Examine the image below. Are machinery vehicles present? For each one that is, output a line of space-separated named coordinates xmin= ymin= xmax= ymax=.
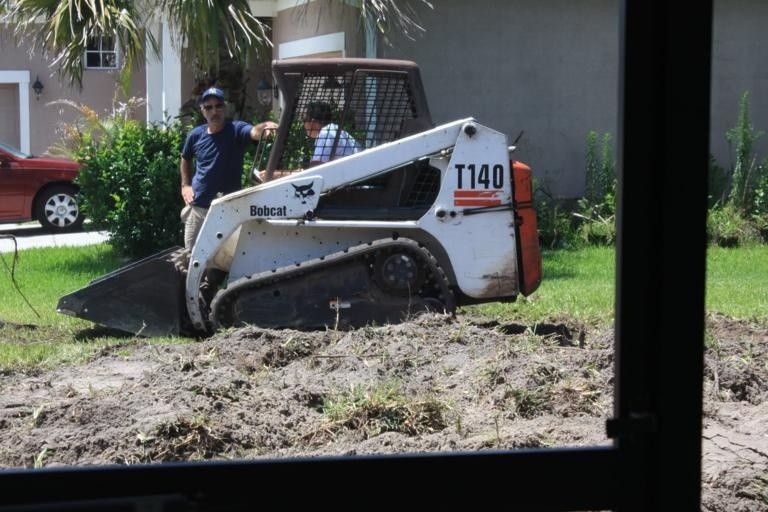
xmin=56 ymin=58 xmax=542 ymax=339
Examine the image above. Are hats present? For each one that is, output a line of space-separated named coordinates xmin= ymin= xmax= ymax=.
xmin=200 ymin=87 xmax=224 ymax=100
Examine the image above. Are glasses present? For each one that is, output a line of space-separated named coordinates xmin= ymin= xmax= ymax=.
xmin=202 ymin=103 xmax=225 ymax=111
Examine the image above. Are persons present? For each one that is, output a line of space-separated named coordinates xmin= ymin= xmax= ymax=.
xmin=259 ymin=102 xmax=364 ymax=179
xmin=180 ymin=87 xmax=279 ymax=251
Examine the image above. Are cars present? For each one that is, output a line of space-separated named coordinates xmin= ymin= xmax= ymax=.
xmin=0 ymin=143 xmax=90 ymax=233
